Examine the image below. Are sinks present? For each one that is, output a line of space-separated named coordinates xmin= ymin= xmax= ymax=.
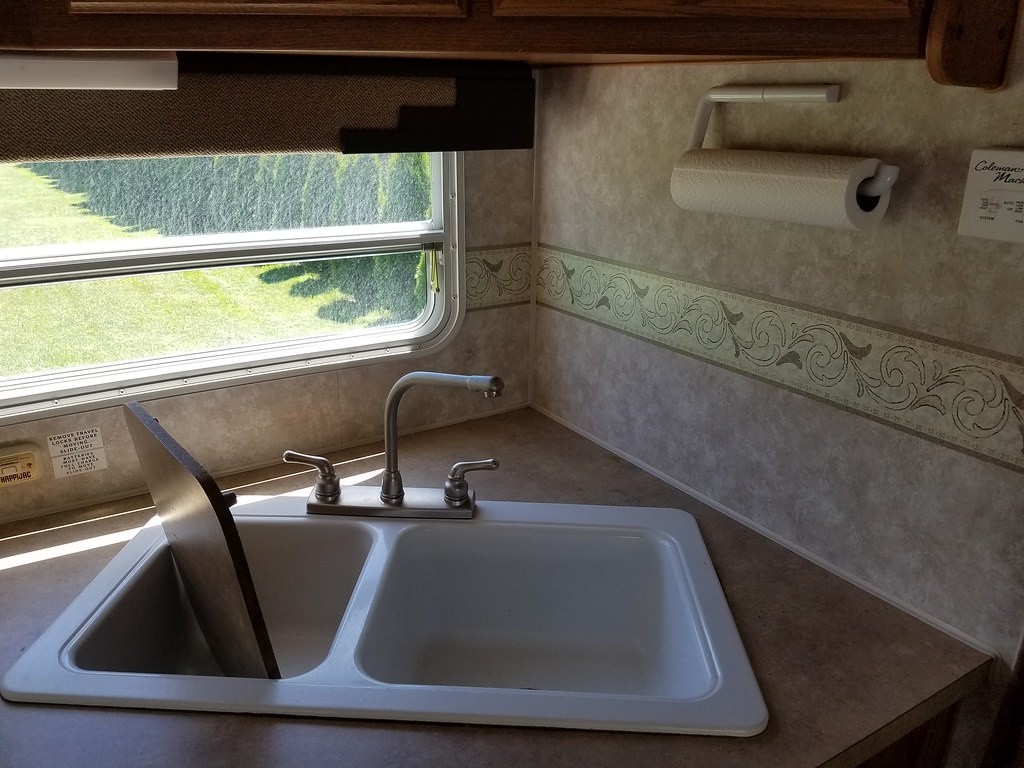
xmin=1 ymin=491 xmax=378 ymax=721
xmin=351 ymin=483 xmax=772 ymax=740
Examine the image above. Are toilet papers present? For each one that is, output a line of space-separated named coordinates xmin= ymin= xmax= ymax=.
xmin=668 ymin=147 xmax=895 ymax=234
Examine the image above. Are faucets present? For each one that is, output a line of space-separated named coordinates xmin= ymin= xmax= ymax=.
xmin=378 ymin=371 xmax=506 ymax=506
